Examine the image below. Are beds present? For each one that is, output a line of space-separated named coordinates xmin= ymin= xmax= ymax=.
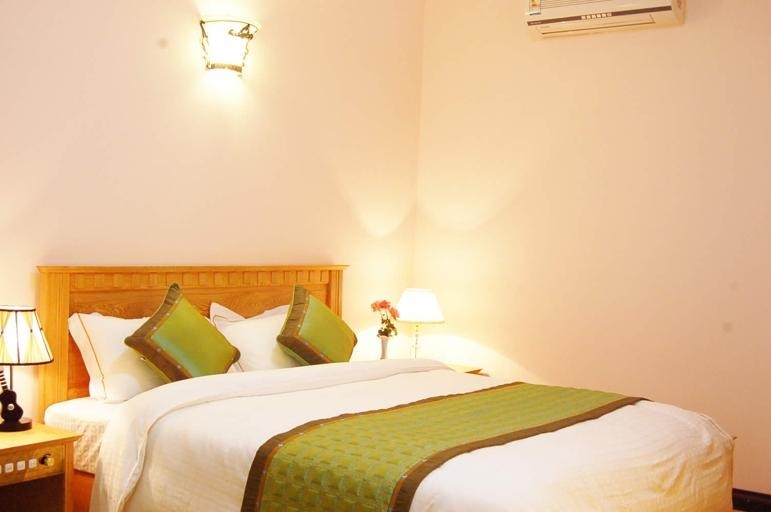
xmin=28 ymin=265 xmax=734 ymax=512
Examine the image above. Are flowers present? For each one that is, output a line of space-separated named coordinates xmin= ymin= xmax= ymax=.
xmin=371 ymin=300 xmax=400 ymax=338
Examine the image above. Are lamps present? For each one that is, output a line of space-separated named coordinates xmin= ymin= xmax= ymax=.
xmin=200 ymin=0 xmax=259 ymax=75
xmin=0 ymin=307 xmax=54 ymax=432
xmin=395 ymin=287 xmax=445 ymax=359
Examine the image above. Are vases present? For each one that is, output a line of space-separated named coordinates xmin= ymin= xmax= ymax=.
xmin=380 ymin=335 xmax=389 ymax=359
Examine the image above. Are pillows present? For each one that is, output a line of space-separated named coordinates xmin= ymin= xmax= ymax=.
xmin=277 ymin=285 xmax=358 ymax=366
xmin=68 ymin=312 xmax=240 ymax=402
xmin=123 ymin=281 xmax=240 ymax=385
xmin=208 ymin=301 xmax=293 ymax=373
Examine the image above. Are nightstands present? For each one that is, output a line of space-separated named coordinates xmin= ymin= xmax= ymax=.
xmin=0 ymin=421 xmax=82 ymax=512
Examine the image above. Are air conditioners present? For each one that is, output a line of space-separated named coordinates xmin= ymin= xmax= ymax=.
xmin=524 ymin=1 xmax=686 ymax=41
